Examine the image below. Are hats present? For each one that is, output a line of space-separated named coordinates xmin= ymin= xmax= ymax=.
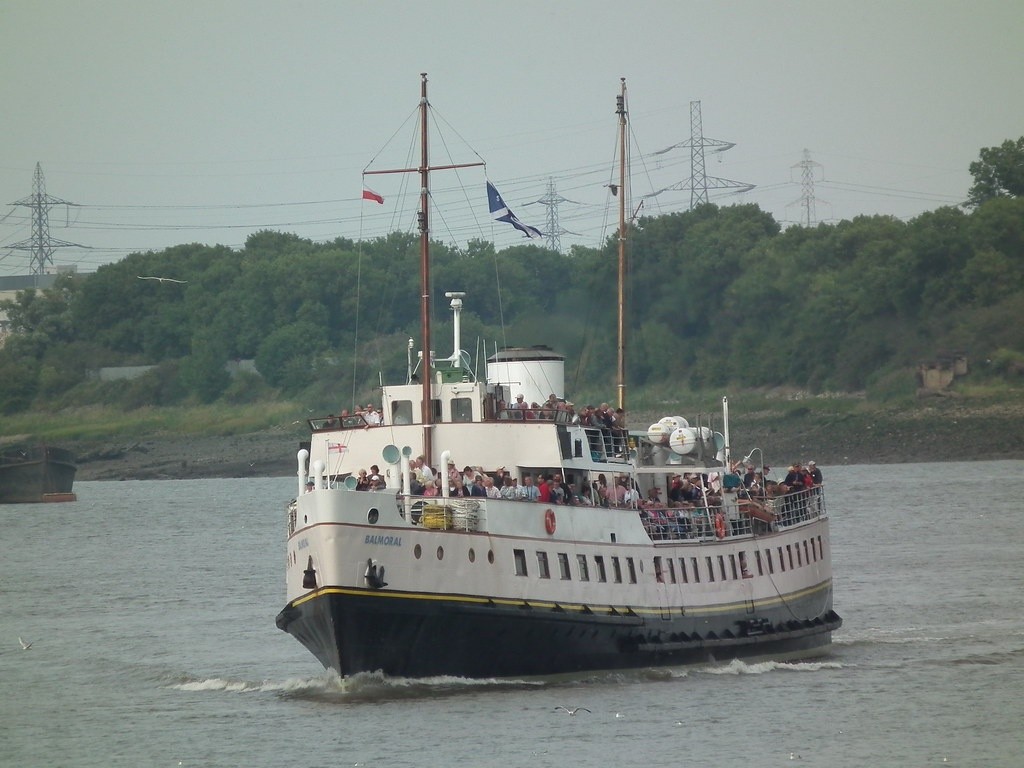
xmin=672 ymin=472 xmax=681 ymax=478
xmin=515 ymin=393 xmax=523 ymax=398
xmin=566 ymin=402 xmax=574 ymax=407
xmin=448 ymin=460 xmax=454 ymax=465
xmin=512 ymin=476 xmax=517 ymax=481
xmin=808 ymin=461 xmax=816 ymax=465
xmin=616 ymin=408 xmax=623 ymax=413
xmin=305 ymin=481 xmax=314 ymax=486
xmin=764 ymin=465 xmax=770 ymax=471
xmin=371 ymin=476 xmax=379 ymax=480
xmin=497 ymin=465 xmax=505 ymax=471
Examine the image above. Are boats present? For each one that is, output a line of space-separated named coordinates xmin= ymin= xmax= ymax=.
xmin=0 ymin=444 xmax=77 ymax=504
xmin=275 ymin=72 xmax=843 ymax=679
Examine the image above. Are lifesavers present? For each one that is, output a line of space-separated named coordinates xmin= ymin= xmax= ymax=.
xmin=546 ymin=509 xmax=556 ymax=534
xmin=715 ymin=513 xmax=725 ymax=539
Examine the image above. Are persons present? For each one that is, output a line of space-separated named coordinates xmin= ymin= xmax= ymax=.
xmin=638 ymin=461 xmax=823 ymax=540
xmin=356 ymin=465 xmax=386 ymax=492
xmin=409 ymin=456 xmax=442 ymax=496
xmin=323 ymin=404 xmax=383 ymax=430
xmin=498 ymin=393 xmax=625 ymax=431
xmin=448 ymin=460 xmax=639 ymax=509
xmin=305 ymin=482 xmax=314 ymax=492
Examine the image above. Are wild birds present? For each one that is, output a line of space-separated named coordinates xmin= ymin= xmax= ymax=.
xmin=18 ymin=637 xmax=33 ymax=651
xmin=603 ymin=183 xmax=622 ymax=196
xmin=675 ymin=721 xmax=685 ymax=725
xmin=554 ymin=704 xmax=592 ymax=716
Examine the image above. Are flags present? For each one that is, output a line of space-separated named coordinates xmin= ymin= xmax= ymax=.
xmin=363 ymin=183 xmax=384 ymax=204
xmin=487 ymin=178 xmax=542 ymax=238
